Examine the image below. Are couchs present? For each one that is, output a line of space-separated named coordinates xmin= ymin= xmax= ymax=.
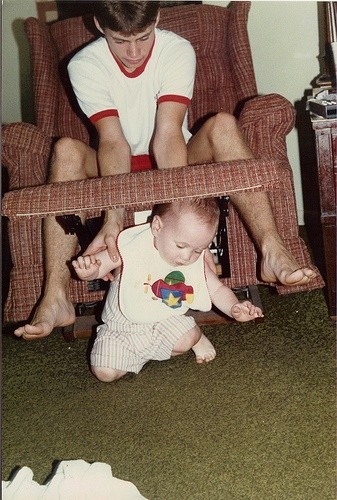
xmin=0 ymin=0 xmax=326 ymax=343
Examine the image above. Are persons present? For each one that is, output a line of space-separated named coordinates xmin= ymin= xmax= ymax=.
xmin=72 ymin=197 xmax=265 ymax=382
xmin=13 ymin=0 xmax=317 ymax=339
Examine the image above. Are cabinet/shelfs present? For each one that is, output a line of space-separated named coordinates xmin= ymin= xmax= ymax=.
xmin=296 ymin=112 xmax=337 ymax=316
